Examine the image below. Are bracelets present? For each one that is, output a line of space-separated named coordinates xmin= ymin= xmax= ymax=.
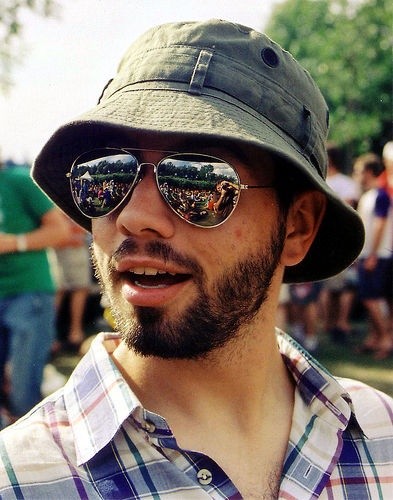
xmin=16 ymin=233 xmax=28 ymax=253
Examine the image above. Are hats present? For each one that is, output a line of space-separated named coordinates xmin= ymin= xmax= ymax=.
xmin=30 ymin=16 xmax=368 ymax=281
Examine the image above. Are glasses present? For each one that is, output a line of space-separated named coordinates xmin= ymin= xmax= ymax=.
xmin=66 ymin=146 xmax=301 ymax=228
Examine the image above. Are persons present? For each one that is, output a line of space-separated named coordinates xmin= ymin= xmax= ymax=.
xmin=277 ymin=139 xmax=393 ymax=359
xmin=44 ymin=213 xmax=94 ymax=357
xmin=0 ymin=18 xmax=393 ymax=500
xmin=71 ymin=172 xmax=239 ymax=226
xmin=0 ymin=158 xmax=71 ymax=422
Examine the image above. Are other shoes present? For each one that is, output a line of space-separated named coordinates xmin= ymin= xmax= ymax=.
xmin=301 ymin=327 xmax=392 ymax=360
